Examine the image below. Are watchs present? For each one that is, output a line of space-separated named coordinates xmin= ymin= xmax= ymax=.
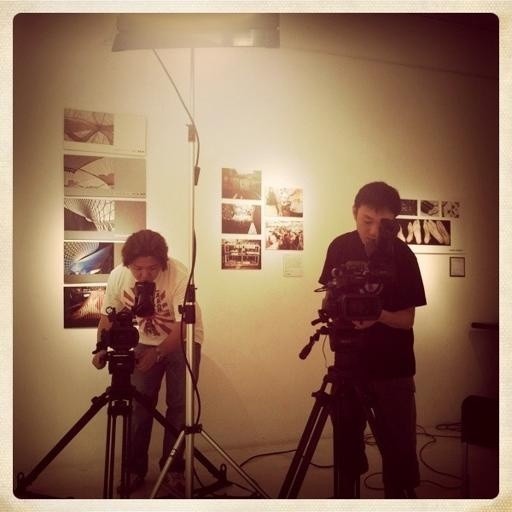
xmin=155 ymin=347 xmax=163 ymax=363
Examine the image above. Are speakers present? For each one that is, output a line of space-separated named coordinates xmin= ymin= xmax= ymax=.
xmin=461 ymin=395 xmax=499 ymax=444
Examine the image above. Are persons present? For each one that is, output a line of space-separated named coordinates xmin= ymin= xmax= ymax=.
xmin=315 ymin=175 xmax=428 ymax=499
xmin=89 ymin=228 xmax=203 ymax=495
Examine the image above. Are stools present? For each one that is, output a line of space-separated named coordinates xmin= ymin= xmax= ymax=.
xmin=461 ymin=396 xmax=499 ymax=498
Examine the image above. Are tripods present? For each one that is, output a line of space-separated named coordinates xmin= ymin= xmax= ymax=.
xmin=278 ymin=323 xmax=418 ymax=499
xmin=14 ymin=350 xmax=231 ymax=497
xmin=150 ymin=46 xmax=269 ymax=499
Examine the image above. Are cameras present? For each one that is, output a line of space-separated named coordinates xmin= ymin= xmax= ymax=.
xmin=89 ymin=280 xmax=157 ymax=353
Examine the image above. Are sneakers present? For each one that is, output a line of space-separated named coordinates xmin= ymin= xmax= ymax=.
xmin=116 ymin=472 xmax=144 ymax=493
xmin=164 ymin=473 xmax=185 ymax=495
